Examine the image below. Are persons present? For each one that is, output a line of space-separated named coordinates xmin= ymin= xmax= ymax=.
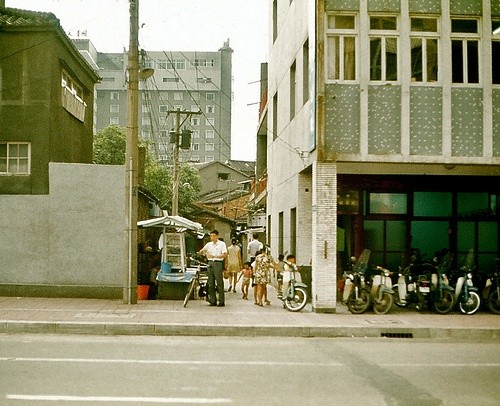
xmin=284 ymin=255 xmax=298 ymax=271
xmin=247 ymin=232 xmax=263 ymax=263
xmin=225 ymin=237 xmax=242 ymax=293
xmin=273 ymin=254 xmax=286 ymax=292
xmin=433 ymin=248 xmax=449 ymax=266
xmin=235 ymin=261 xmax=253 ymax=300
xmin=411 ymin=248 xmax=421 ymax=274
xmin=251 ymin=245 xmax=283 ymax=305
xmin=200 ymin=230 xmax=228 ymax=307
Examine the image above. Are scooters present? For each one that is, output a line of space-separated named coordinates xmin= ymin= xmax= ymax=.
xmin=278 ymin=260 xmax=308 ymax=312
xmin=342 ymin=256 xmax=500 ymax=316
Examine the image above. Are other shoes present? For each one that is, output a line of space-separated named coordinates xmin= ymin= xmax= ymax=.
xmin=218 ymin=303 xmax=225 ymax=307
xmin=209 ymin=303 xmax=217 ymax=306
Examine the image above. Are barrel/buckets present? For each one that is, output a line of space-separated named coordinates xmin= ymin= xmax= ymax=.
xmin=161 ymin=261 xmax=172 ymax=274
xmin=137 ymin=285 xmax=150 ymax=300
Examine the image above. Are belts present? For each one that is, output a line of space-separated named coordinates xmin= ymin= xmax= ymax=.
xmin=208 ymin=260 xmax=222 ymax=263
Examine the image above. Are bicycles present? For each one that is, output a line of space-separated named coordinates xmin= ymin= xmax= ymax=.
xmin=183 ymin=257 xmax=221 ymax=308
